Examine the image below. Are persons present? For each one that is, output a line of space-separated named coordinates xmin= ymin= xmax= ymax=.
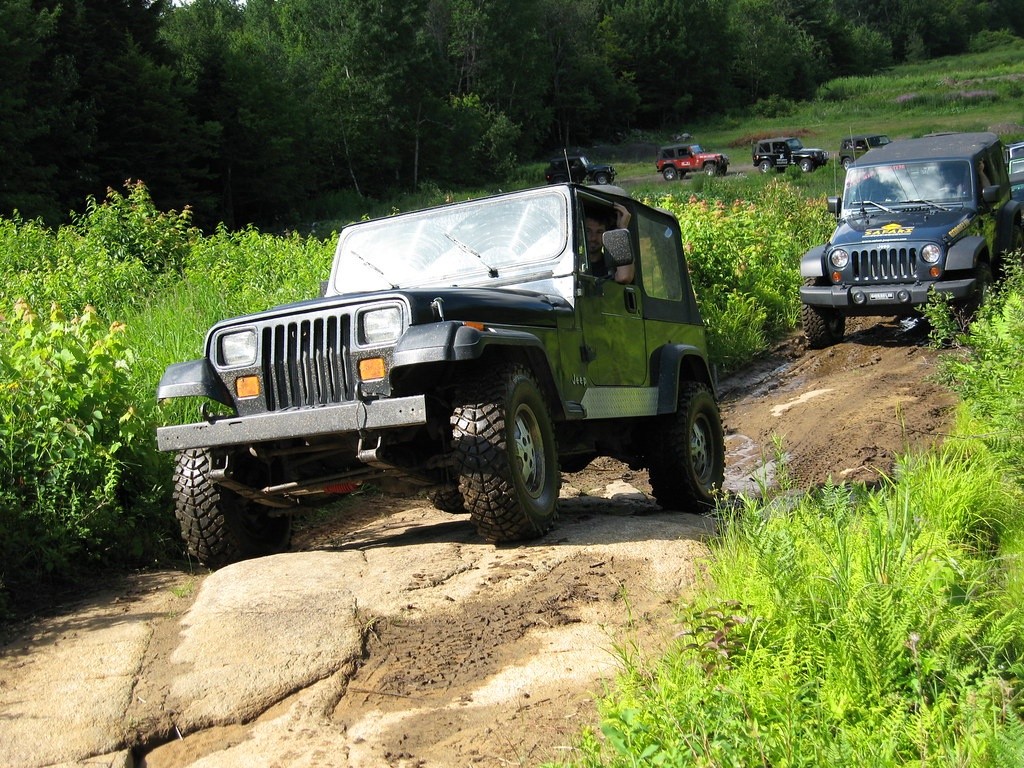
xmin=583 ymin=201 xmax=637 ymax=285
xmin=976 ymin=159 xmax=991 ymax=187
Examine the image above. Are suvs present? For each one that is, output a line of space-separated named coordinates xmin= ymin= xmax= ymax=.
xmin=751 ymin=137 xmax=830 ymax=175
xmin=655 ymin=144 xmax=731 ymax=181
xmin=544 ymin=155 xmax=618 ymax=185
xmin=153 ymin=179 xmax=730 ymax=571
xmin=838 ymin=134 xmax=894 ymax=174
xmin=920 ymin=131 xmax=1024 ymax=225
xmin=798 ymin=132 xmax=1024 ymax=348
